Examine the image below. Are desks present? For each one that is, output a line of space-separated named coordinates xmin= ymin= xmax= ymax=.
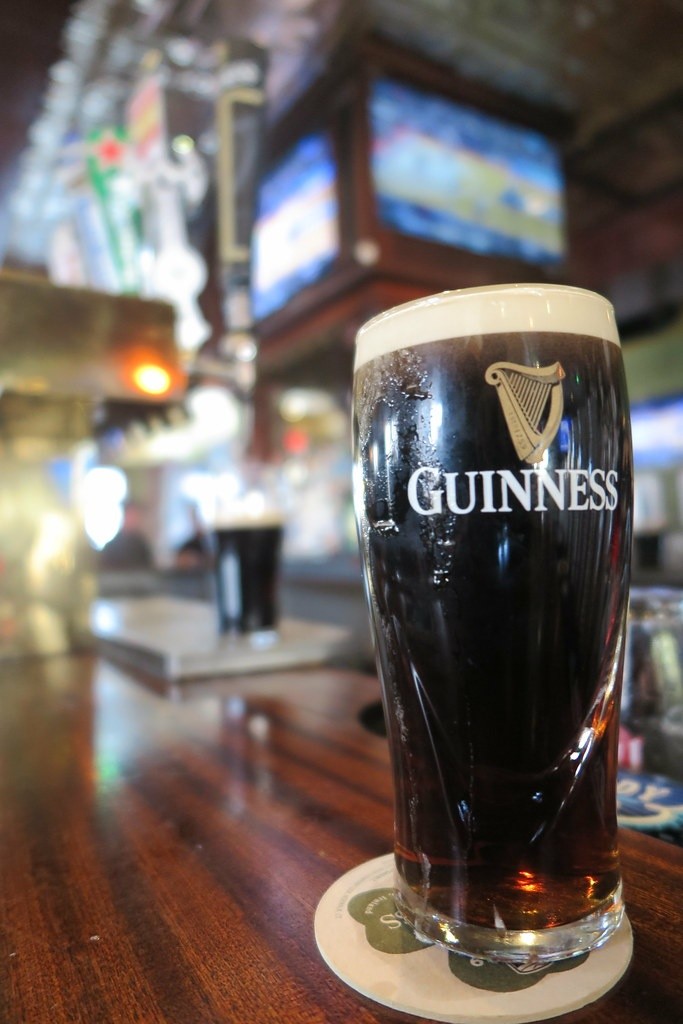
xmin=1 ymin=655 xmax=683 ymax=1024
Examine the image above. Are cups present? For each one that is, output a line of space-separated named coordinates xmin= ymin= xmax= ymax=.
xmin=352 ymin=280 xmax=636 ymax=970
xmin=200 ymin=465 xmax=287 ymax=647
